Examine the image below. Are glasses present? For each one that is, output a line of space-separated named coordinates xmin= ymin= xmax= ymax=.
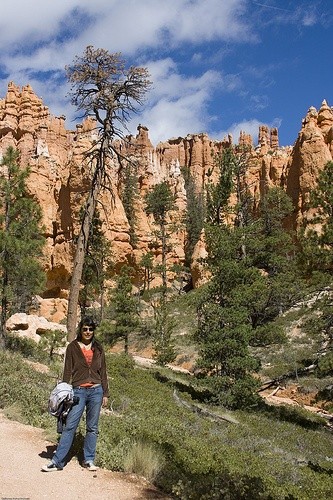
xmin=81 ymin=327 xmax=94 ymax=333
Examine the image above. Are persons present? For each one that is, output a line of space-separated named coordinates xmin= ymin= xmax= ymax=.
xmin=40 ymin=317 xmax=110 ymax=472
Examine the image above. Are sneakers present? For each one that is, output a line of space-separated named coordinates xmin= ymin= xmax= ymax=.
xmin=42 ymin=461 xmax=64 ymax=472
xmin=84 ymin=461 xmax=98 ymax=471
